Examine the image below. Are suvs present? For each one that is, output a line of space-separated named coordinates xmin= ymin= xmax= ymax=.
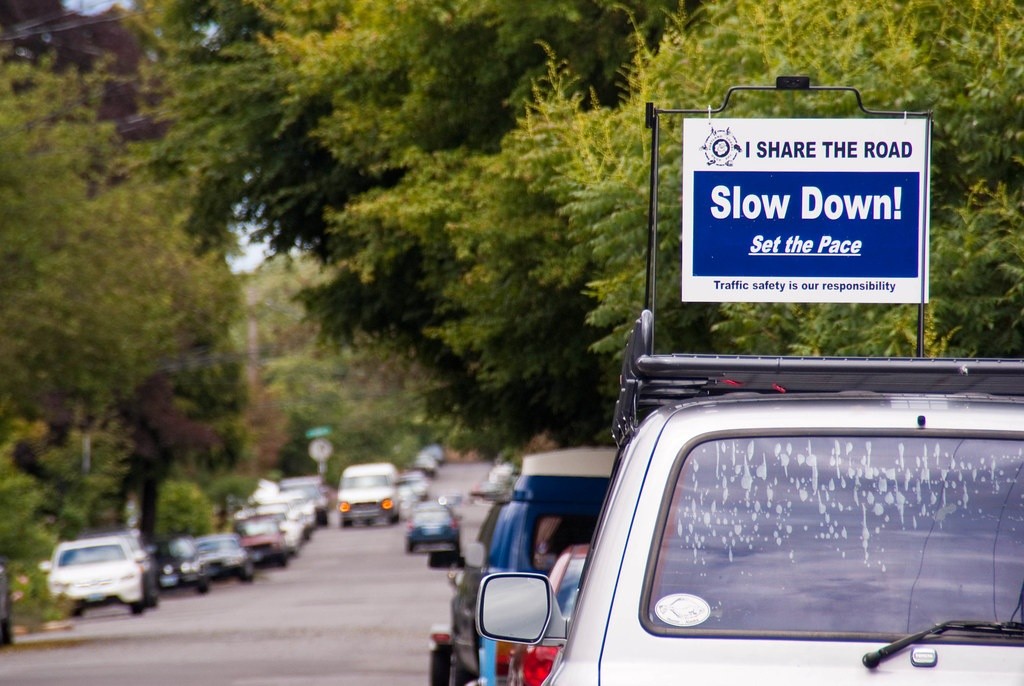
xmin=253 ymin=478 xmax=327 ymax=557
xmin=39 ymin=527 xmax=157 ymax=617
xmin=235 ymin=518 xmax=288 ymax=568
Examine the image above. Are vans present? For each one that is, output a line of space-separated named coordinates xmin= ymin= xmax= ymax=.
xmin=449 ymin=446 xmax=619 ymax=686
xmin=336 ymin=463 xmax=399 ymax=527
xmin=478 ymin=307 xmax=1024 ymax=686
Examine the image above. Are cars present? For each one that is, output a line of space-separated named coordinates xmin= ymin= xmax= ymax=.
xmin=405 ymin=502 xmax=458 ymax=552
xmin=146 ymin=533 xmax=208 ymax=593
xmin=193 ymin=535 xmax=253 ymax=582
xmin=398 ymin=442 xmax=443 ymax=518
xmin=507 ymin=544 xmax=589 ymax=686
